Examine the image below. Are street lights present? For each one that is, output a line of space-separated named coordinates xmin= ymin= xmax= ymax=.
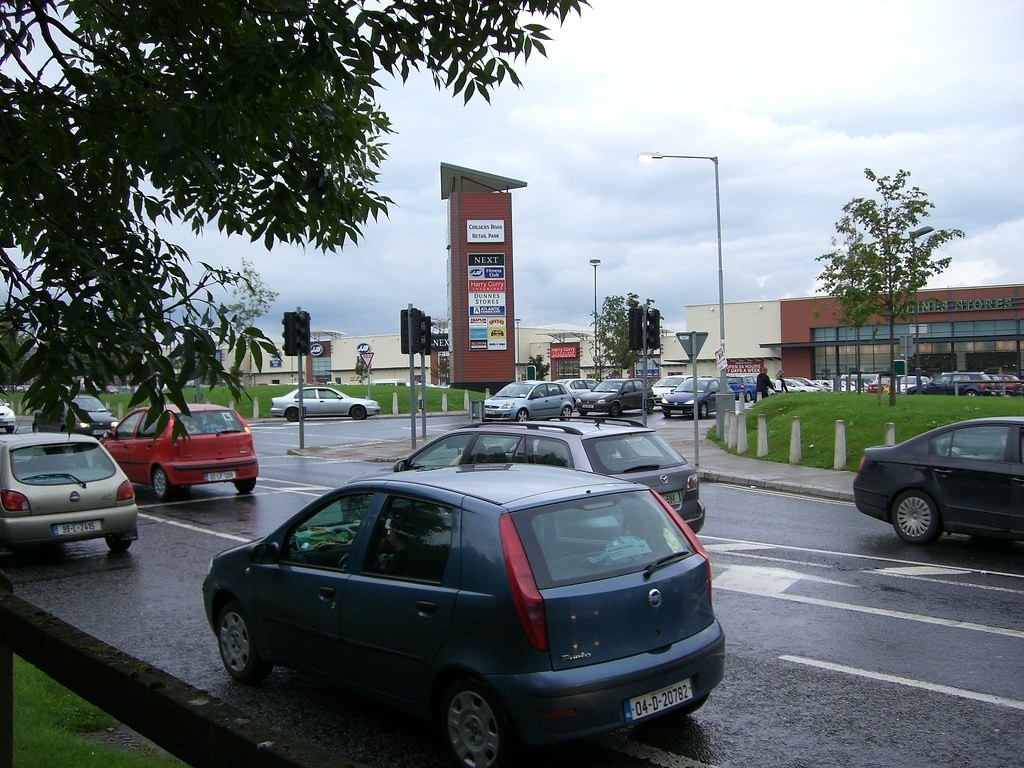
xmin=909 ymin=227 xmax=934 ymax=391
xmin=589 ymin=259 xmax=601 ymax=381
xmin=515 ymin=318 xmax=521 ymax=381
xmin=637 ymin=152 xmax=726 ymax=393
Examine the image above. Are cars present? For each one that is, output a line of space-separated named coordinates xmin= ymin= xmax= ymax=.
xmin=483 ymin=379 xmax=574 ymax=423
xmin=99 ymin=404 xmax=258 ymax=501
xmin=0 ymin=432 xmax=138 ymax=550
xmin=907 ymin=371 xmax=996 ymax=396
xmin=270 ymin=387 xmax=381 ymax=420
xmin=204 ymin=464 xmax=724 ymax=768
xmin=988 ymin=375 xmax=1024 ymax=396
xmin=0 ymin=398 xmax=16 ymax=432
xmin=771 ymin=375 xmax=930 ymax=396
xmin=3 ymin=380 xmax=140 ymax=393
xmin=853 ymin=416 xmax=1024 ymax=545
xmin=32 ymin=394 xmax=119 ymax=439
xmin=552 ymin=376 xmax=756 ymax=422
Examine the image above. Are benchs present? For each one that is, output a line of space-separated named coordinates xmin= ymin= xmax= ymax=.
xmin=940 ymin=438 xmax=961 ymax=456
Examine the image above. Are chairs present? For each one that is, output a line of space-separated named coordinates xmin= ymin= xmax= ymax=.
xmin=484 ymin=446 xmax=507 ymax=463
xmin=537 ymin=440 xmax=557 ymax=456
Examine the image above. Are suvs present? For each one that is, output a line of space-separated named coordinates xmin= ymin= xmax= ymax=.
xmin=341 ymin=415 xmax=705 ymax=543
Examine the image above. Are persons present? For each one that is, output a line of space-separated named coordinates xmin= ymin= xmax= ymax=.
xmin=377 ymin=516 xmax=415 ymax=576
xmin=773 ymin=370 xmax=789 ymax=394
xmin=754 ymin=367 xmax=774 ymax=403
xmin=448 ymin=442 xmax=486 ymax=465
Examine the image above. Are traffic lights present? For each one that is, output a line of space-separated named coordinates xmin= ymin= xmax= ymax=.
xmin=646 ymin=308 xmax=660 ymax=350
xmin=296 ymin=311 xmax=310 ymax=354
